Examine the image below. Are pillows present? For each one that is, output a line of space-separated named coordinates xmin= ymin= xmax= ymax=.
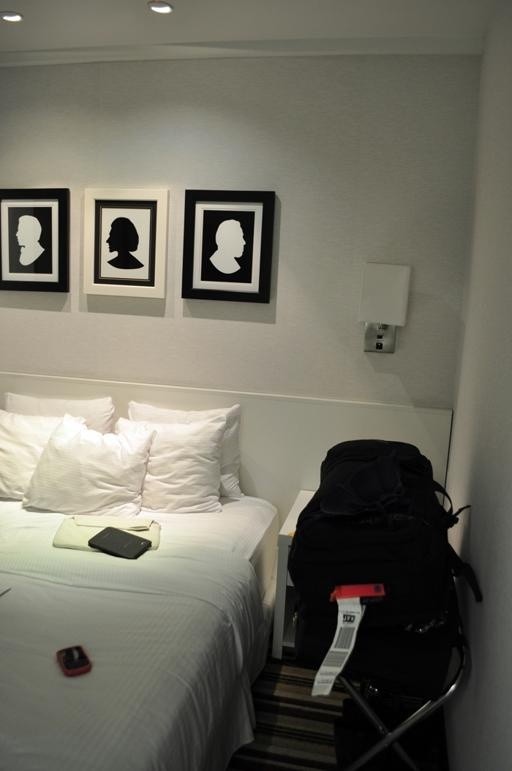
xmin=0 ymin=398 xmax=247 ymax=519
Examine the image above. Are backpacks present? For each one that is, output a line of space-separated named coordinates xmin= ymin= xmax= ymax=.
xmin=288 ymin=440 xmax=462 ymax=698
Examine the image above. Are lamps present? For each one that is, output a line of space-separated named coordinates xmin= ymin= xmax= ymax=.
xmin=357 ymin=262 xmax=413 ymax=354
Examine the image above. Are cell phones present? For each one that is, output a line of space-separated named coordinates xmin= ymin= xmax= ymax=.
xmin=57 ymin=646 xmax=92 ymax=676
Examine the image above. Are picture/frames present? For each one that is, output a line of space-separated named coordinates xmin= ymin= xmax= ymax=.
xmin=181 ymin=189 xmax=275 ymax=304
xmin=82 ymin=187 xmax=170 ymax=300
xmin=0 ymin=187 xmax=71 ymax=294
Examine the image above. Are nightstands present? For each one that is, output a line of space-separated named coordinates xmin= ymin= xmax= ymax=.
xmin=273 ymin=488 xmax=318 ymax=660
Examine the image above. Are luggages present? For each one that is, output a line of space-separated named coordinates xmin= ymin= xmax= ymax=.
xmin=333 ymin=682 xmax=448 ymax=771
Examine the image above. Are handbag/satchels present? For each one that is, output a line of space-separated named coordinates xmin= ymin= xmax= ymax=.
xmin=53 ymin=516 xmax=160 ymax=552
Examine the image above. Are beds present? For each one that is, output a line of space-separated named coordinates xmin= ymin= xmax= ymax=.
xmin=0 ymin=495 xmax=281 ymax=771
